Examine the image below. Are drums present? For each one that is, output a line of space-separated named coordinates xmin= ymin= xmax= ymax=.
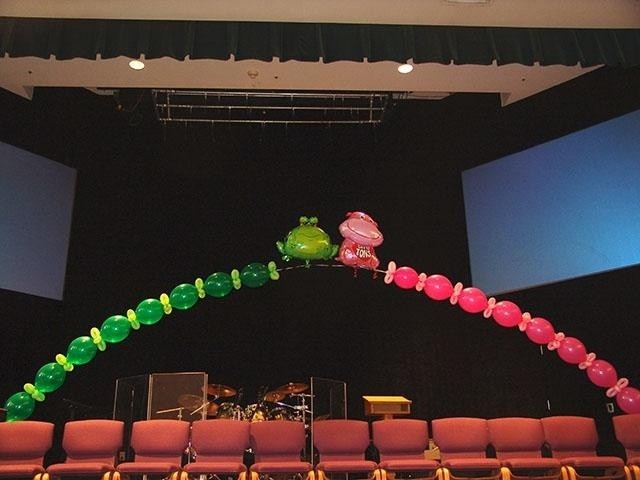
xmin=246 ymin=404 xmax=268 ymax=421
xmin=217 ymin=403 xmax=245 ymax=419
xmin=270 ymin=408 xmax=291 ymax=420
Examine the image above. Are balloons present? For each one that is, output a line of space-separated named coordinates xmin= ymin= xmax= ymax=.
xmin=275 ymin=216 xmax=339 ymax=268
xmin=2 ymin=262 xmax=279 ymax=424
xmin=384 ymin=261 xmax=640 ymax=413
xmin=334 ymin=211 xmax=384 ymax=280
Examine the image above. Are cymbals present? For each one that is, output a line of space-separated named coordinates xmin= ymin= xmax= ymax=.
xmin=201 ymin=384 xmax=236 ymax=397
xmin=279 ymin=384 xmax=308 ymax=392
xmin=179 ymin=395 xmax=218 ymax=416
xmin=263 ymin=392 xmax=285 ymax=401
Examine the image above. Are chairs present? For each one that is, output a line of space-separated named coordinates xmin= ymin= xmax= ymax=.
xmin=371 ymin=418 xmax=444 ymax=479
xmin=541 ymin=416 xmax=632 ymax=478
xmin=432 ymin=417 xmax=506 ymax=479
xmin=610 ymin=413 xmax=638 ymax=479
xmin=1 ymin=420 xmax=56 ymax=479
xmin=180 ymin=419 xmax=250 ymax=478
xmin=42 ymin=420 xmax=124 ymax=479
xmin=249 ymin=421 xmax=316 ymax=480
xmin=112 ymin=420 xmax=190 ymax=479
xmin=489 ymin=416 xmax=569 ymax=479
xmin=310 ymin=420 xmax=382 ymax=478
